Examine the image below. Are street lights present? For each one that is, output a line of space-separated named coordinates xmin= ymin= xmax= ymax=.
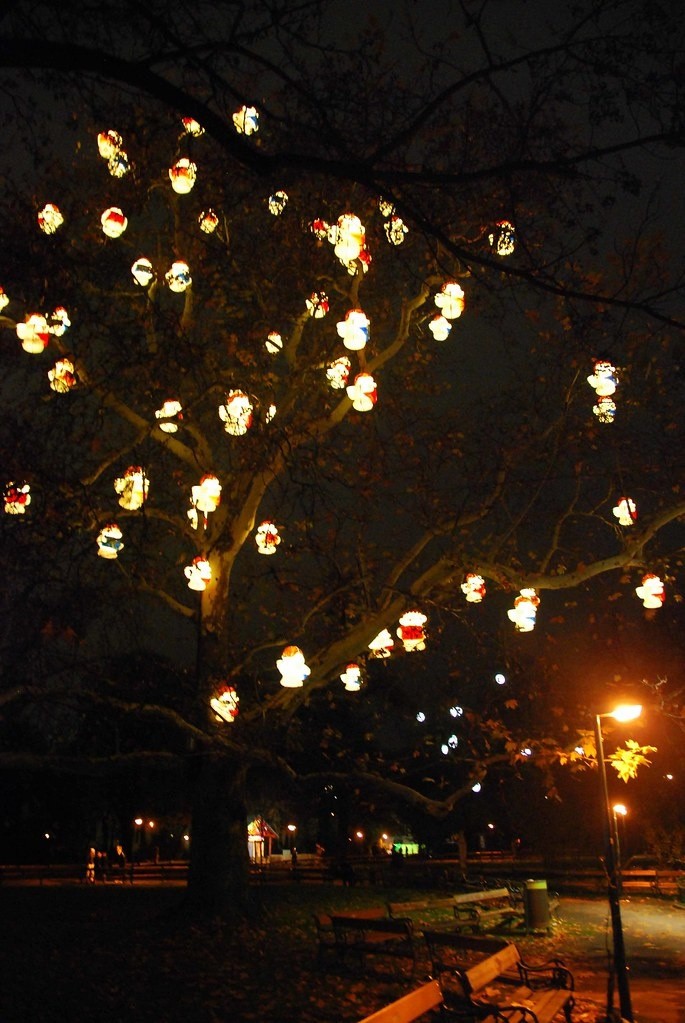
xmin=129 ymin=817 xmax=143 ymax=884
xmin=287 ymin=824 xmax=298 ymax=869
xmin=597 ymin=702 xmax=648 ymax=1023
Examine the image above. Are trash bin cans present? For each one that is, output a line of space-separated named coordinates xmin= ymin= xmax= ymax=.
xmin=522 ymin=879 xmax=553 ymax=928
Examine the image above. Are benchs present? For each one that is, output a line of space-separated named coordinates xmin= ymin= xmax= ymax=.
xmin=325 ymin=912 xmax=441 ymax=986
xmin=449 ymin=886 xmax=529 ymax=940
xmin=384 ymin=897 xmax=479 ymax=958
xmin=424 ymin=931 xmax=576 ymax=1023
